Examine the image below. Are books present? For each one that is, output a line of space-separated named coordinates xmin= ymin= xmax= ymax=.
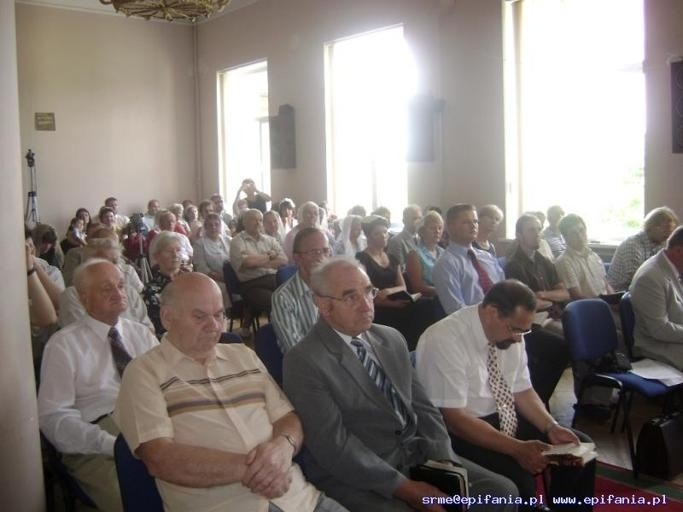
xmin=411 ymin=458 xmax=470 ymax=511
xmin=542 ymin=440 xmax=596 ymax=477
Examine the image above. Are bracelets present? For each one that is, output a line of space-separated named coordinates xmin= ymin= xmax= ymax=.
xmin=279 ymin=431 xmax=297 ymax=452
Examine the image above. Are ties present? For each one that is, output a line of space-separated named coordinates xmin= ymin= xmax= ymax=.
xmin=467 ymin=249 xmax=493 ymax=296
xmin=487 ymin=342 xmax=519 ymax=439
xmin=108 ymin=327 xmax=132 ymax=380
xmin=350 ymin=336 xmax=409 ymax=431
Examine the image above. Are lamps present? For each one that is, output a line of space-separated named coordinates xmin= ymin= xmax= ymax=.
xmin=99 ymin=1 xmax=231 ymax=25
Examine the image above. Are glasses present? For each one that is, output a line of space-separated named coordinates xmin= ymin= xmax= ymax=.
xmin=318 ymin=287 xmax=378 ymax=306
xmin=504 ymin=321 xmax=531 ymax=338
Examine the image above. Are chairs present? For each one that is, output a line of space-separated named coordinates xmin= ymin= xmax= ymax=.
xmin=112 ymin=260 xmax=682 ymax=511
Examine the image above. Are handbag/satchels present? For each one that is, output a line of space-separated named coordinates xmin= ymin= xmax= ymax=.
xmin=599 ymin=353 xmax=631 ymax=374
xmin=636 ymin=411 xmax=682 ymax=480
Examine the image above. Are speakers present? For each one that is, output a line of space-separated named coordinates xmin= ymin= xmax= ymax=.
xmin=670 ymin=60 xmax=683 ymax=153
xmin=270 ymin=104 xmax=296 ymax=169
xmin=407 ymin=89 xmax=435 ymax=161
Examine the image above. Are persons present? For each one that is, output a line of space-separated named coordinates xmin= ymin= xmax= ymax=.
xmin=113 ymin=271 xmax=350 ymax=512
xmin=36 ymin=261 xmax=161 ymax=511
xmin=414 ymin=280 xmax=595 ymax=511
xmin=21 ymin=176 xmax=682 ymax=379
xmin=282 ymin=258 xmax=519 ymax=511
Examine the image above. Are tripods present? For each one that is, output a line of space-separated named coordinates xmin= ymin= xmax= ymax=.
xmin=24 ymin=149 xmax=38 ymax=226
xmin=133 ymin=233 xmax=154 ymax=284
